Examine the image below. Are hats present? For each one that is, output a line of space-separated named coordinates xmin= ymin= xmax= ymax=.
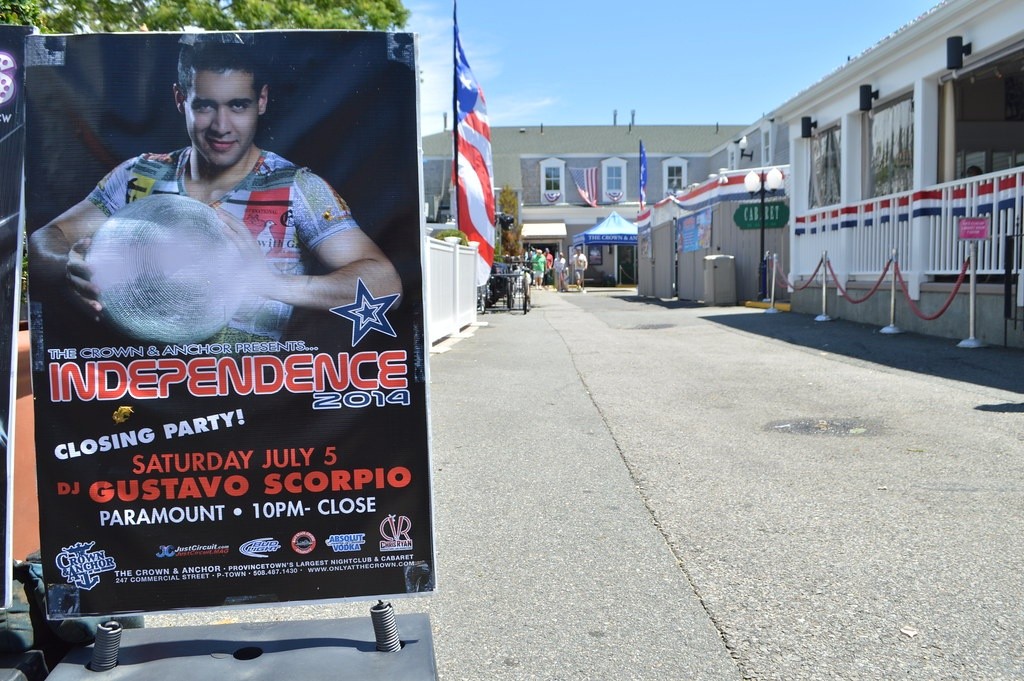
xmin=576 ymin=246 xmax=581 ymax=250
xmin=545 ymin=248 xmax=549 ymax=252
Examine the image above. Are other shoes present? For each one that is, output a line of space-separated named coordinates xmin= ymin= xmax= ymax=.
xmin=535 ymin=286 xmax=542 ymax=290
xmin=577 ymin=286 xmax=583 ymax=292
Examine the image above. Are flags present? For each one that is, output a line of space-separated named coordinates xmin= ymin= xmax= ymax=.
xmin=446 ymin=1 xmax=499 ymax=269
xmin=567 ymin=166 xmax=600 ymax=209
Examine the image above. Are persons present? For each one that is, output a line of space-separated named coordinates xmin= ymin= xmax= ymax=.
xmin=571 ymin=247 xmax=588 ymax=292
xmin=529 ymin=246 xmax=553 ymax=290
xmin=554 ymin=252 xmax=566 ymax=292
xmin=27 ymin=35 xmax=406 ymax=316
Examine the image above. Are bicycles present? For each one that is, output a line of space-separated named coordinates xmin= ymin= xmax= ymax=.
xmin=478 ymin=286 xmax=487 ymax=314
xmin=522 ymin=260 xmax=538 ymax=314
xmin=501 ymin=261 xmax=520 ymax=308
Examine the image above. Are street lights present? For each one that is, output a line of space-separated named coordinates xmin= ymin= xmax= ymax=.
xmin=744 ymin=169 xmax=783 ymax=302
